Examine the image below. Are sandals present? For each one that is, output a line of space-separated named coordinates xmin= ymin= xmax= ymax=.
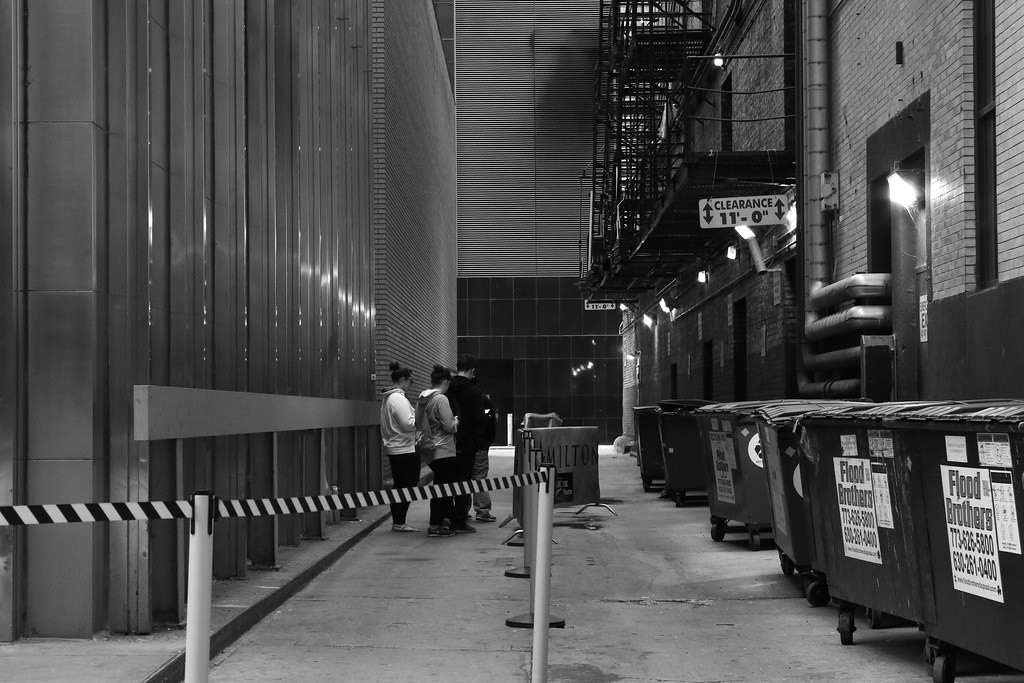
xmin=392 ymin=522 xmax=421 ymax=532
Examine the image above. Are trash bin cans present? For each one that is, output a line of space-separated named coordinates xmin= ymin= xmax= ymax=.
xmin=690 ymin=396 xmax=861 ymax=530
xmin=656 ymin=399 xmax=721 ymax=504
xmin=798 ymin=398 xmax=1024 ymax=673
xmin=750 ymin=401 xmax=912 ymax=572
xmin=631 ymin=405 xmax=664 ymax=493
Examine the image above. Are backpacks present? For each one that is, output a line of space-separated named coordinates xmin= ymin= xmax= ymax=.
xmin=414 ymin=393 xmax=454 ymax=453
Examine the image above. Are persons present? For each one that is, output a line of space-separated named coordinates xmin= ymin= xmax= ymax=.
xmin=379 ymin=361 xmax=422 ymax=531
xmin=414 ymin=363 xmax=497 ymax=537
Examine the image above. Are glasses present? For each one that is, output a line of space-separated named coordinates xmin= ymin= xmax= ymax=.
xmin=403 ymin=376 xmax=413 ymax=385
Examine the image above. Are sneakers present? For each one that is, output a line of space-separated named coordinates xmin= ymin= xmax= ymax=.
xmin=451 ymin=522 xmax=476 ymax=533
xmin=466 ymin=515 xmax=472 ymax=520
xmin=427 ymin=527 xmax=456 ymax=537
xmin=476 ymin=512 xmax=497 ymax=522
